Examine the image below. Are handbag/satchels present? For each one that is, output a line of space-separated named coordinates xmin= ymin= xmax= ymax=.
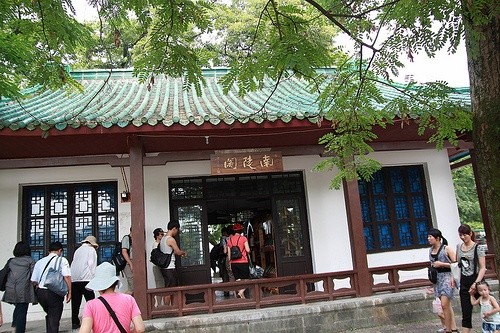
xmin=44 ymin=257 xmax=68 ymax=296
xmin=428 ymin=267 xmax=437 ymax=283
xmin=0 ymin=258 xmax=13 ymax=291
xmin=112 ymin=235 xmax=132 ymax=270
xmin=150 ymin=248 xmax=171 ymax=268
xmin=432 ymin=297 xmax=444 ymax=318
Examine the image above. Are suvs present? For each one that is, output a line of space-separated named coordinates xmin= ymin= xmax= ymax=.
xmin=474 ymin=239 xmax=488 ymax=251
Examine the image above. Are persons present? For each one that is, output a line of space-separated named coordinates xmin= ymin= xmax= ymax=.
xmin=456 ymin=224 xmax=486 ymax=333
xmin=1 ymin=241 xmax=38 ymax=333
xmin=78 ymin=262 xmax=145 ymax=333
xmin=70 ymin=236 xmax=99 ymax=329
xmin=123 ymin=227 xmax=134 ymax=295
xmin=469 ymin=281 xmax=500 ymax=333
xmin=152 ymin=228 xmax=168 ymax=306
xmin=218 ymin=223 xmax=252 ymax=299
xmin=427 ymin=228 xmax=460 ymax=333
xmin=159 ymin=219 xmax=187 ymax=306
xmin=30 ymin=241 xmax=72 ymax=333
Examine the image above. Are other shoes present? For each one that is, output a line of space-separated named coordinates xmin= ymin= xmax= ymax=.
xmin=237 ymin=292 xmax=245 ymax=299
xmin=437 ymin=327 xmax=446 ymax=333
xmin=72 ymin=324 xmax=81 ymax=329
xmin=447 ymin=330 xmax=460 ymax=333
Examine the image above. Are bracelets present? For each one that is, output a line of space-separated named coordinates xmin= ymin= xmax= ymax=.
xmin=474 ymin=282 xmax=476 ymax=285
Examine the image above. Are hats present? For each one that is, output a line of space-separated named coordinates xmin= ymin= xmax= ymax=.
xmin=81 ymin=236 xmax=99 ymax=250
xmin=84 ymin=262 xmax=122 ymax=291
xmin=233 ymin=223 xmax=243 ymax=230
xmin=153 ymin=228 xmax=168 ymax=233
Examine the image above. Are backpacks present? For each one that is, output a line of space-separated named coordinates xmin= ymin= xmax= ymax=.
xmin=231 ymin=236 xmax=245 ymax=260
